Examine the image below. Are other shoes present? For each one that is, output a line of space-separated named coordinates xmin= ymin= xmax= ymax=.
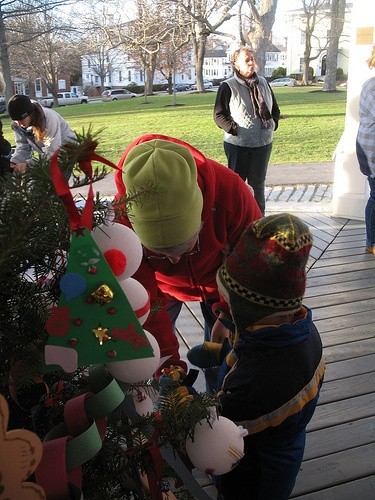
xmin=366 ymin=245 xmax=375 ymax=254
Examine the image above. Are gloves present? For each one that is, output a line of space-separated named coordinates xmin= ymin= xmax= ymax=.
xmin=187 ymin=342 xmax=224 ymax=368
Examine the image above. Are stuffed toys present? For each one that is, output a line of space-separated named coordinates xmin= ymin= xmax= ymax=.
xmin=91 ymin=221 xmax=160 ymax=385
xmin=185 ymin=417 xmax=248 ymax=476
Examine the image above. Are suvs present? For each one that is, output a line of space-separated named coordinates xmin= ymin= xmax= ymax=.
xmin=101 ymin=89 xmax=139 ymax=102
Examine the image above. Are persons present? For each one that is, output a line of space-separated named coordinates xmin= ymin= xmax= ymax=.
xmin=161 ymin=211 xmax=328 ymax=500
xmin=0 ymin=119 xmax=13 ymax=178
xmin=7 ymin=94 xmax=77 ymax=189
xmin=354 ymin=38 xmax=375 ymax=253
xmin=105 ymin=134 xmax=266 ymax=388
xmin=213 ymin=47 xmax=281 ymax=221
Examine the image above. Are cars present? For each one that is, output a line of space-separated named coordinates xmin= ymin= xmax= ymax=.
xmin=166 ymin=83 xmax=189 ymax=92
xmin=268 ymin=78 xmax=296 ymax=87
xmin=191 ymin=79 xmax=213 ymax=90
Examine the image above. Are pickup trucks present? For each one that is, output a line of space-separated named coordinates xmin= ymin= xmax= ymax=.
xmin=42 ymin=92 xmax=88 ymax=108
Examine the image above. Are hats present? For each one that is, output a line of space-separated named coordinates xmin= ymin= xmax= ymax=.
xmin=7 ymin=93 xmax=33 ymax=120
xmin=123 ymin=138 xmax=202 ymax=247
xmin=215 ymin=213 xmax=314 ymax=333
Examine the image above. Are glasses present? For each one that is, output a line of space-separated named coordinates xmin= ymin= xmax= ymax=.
xmin=143 ymin=235 xmax=200 ymax=259
